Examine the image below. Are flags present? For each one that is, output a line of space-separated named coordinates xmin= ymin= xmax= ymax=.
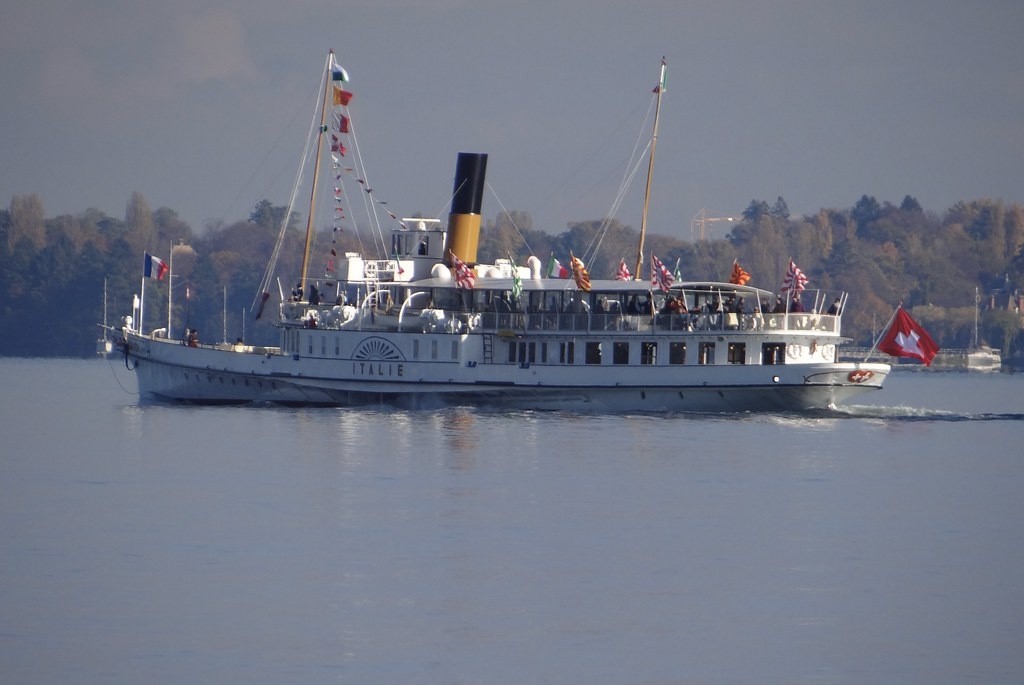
xmin=782 ymin=260 xmax=809 ymax=301
xmin=451 ymin=253 xmax=475 ymax=289
xmin=652 ymin=255 xmax=675 ymax=293
xmin=144 ymin=252 xmax=167 ymax=280
xmin=615 ymin=259 xmax=630 ymax=280
xmin=332 ymin=63 xmax=352 ymax=106
xmin=879 ymin=308 xmax=940 ymax=367
xmin=651 ymin=69 xmax=666 ymax=93
xmin=396 ymin=250 xmax=405 ymax=274
xmin=547 ymin=256 xmax=567 ymax=279
xmin=570 ymin=256 xmax=591 ymax=291
xmin=508 ymin=256 xmax=522 ymax=297
xmin=674 ymin=268 xmax=681 ymax=282
xmin=324 ymin=109 xmax=407 ymax=278
xmin=728 ymin=262 xmax=752 ymax=285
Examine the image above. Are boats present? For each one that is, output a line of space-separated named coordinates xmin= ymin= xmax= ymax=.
xmin=96 ymin=48 xmax=892 ymax=414
xmin=963 ymin=284 xmax=1001 ymax=370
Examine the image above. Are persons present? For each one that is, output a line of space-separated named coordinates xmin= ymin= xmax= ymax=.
xmin=189 ymin=329 xmax=197 ymax=347
xmin=593 ymin=292 xmax=764 ymax=331
xmin=502 ymin=295 xmax=560 ymax=330
xmin=761 ymin=296 xmax=805 ymax=313
xmin=294 ymin=284 xmax=320 ymax=305
xmin=827 ymin=297 xmax=842 ymax=315
